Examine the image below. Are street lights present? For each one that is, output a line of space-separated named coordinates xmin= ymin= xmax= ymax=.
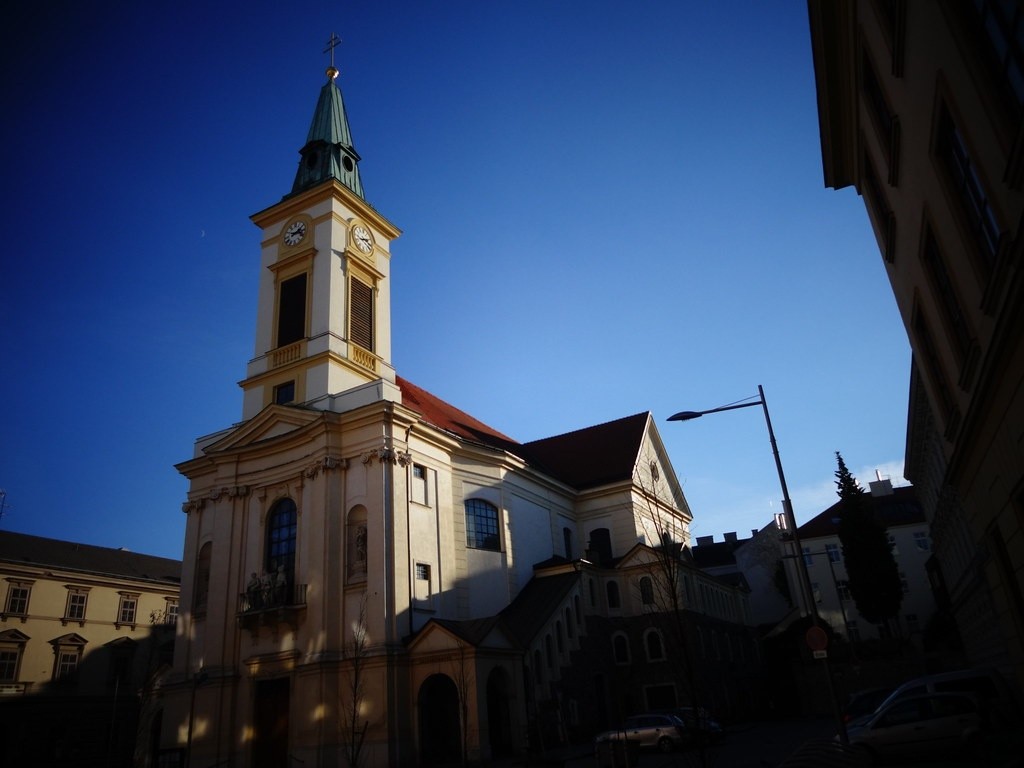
xmin=187 ymin=673 xmax=208 ymax=768
xmin=665 ymin=385 xmax=852 ymax=761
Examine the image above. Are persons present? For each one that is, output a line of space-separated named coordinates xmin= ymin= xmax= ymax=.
xmin=246 ymin=563 xmax=287 ymax=610
xmin=348 ymin=526 xmax=367 ymax=578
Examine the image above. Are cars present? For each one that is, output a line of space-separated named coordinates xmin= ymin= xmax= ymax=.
xmin=837 ymin=693 xmax=1001 ymax=764
xmin=593 ymin=711 xmax=725 ymax=757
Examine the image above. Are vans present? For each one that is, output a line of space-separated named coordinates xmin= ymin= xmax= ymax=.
xmin=837 ymin=670 xmax=1017 ymax=761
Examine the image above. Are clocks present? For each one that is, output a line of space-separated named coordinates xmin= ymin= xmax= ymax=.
xmin=284 ymin=220 xmax=307 ymax=246
xmin=355 ymin=226 xmax=372 ymax=253
